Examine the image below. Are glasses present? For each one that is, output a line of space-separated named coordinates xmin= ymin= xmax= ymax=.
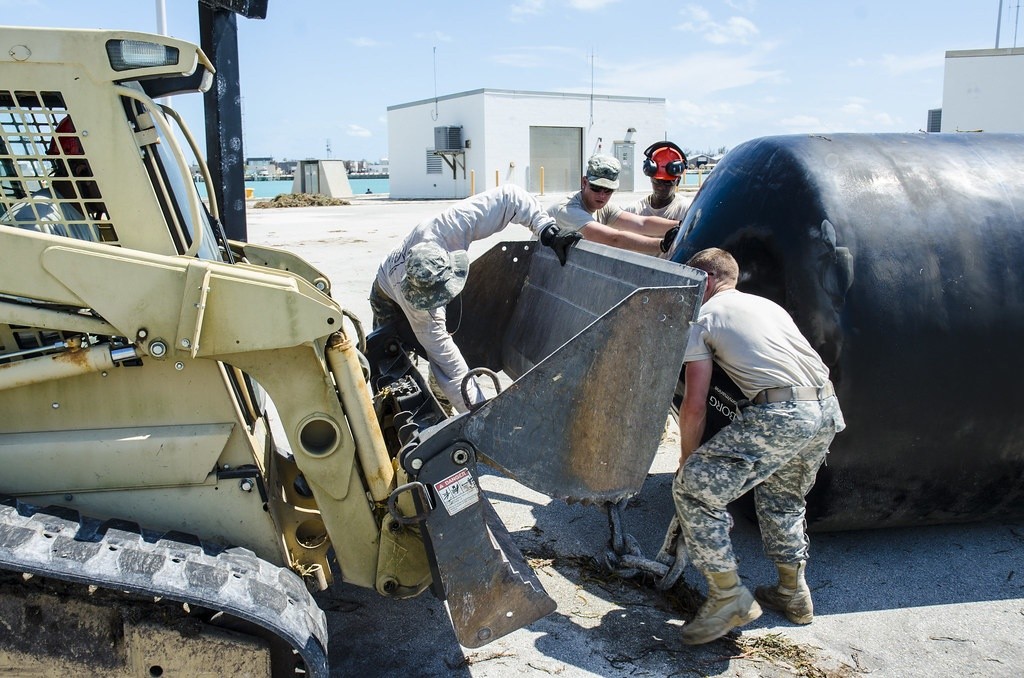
xmin=588 ymin=183 xmax=613 ymax=194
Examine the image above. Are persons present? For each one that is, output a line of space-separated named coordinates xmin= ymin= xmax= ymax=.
xmin=624 ymin=141 xmax=694 ymax=220
xmin=530 ymin=153 xmax=682 ymax=253
xmin=0 ymin=113 xmax=107 ymax=337
xmin=369 ymin=184 xmax=584 ymax=416
xmin=672 ymin=247 xmax=847 ymax=646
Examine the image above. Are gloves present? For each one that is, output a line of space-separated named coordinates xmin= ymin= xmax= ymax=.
xmin=540 ymin=224 xmax=584 ymax=267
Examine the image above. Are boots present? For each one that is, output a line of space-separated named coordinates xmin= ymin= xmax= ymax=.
xmin=677 ymin=570 xmax=762 ymax=645
xmin=754 ymin=562 xmax=813 ymax=624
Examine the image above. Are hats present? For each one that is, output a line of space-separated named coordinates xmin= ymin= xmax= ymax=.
xmin=400 ymin=241 xmax=470 ymax=310
xmin=651 ymin=146 xmax=686 ymax=180
xmin=586 ymin=154 xmax=621 ymax=189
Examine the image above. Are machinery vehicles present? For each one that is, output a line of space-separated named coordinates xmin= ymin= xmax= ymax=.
xmin=2 ymin=2 xmax=711 ymax=678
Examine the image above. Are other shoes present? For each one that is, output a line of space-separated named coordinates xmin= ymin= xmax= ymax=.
xmin=658 ymin=415 xmax=680 ymax=445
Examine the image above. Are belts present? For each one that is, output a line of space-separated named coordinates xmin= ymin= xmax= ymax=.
xmin=753 ymin=381 xmax=834 ymax=406
xmin=374 ymin=279 xmax=390 ymax=301
